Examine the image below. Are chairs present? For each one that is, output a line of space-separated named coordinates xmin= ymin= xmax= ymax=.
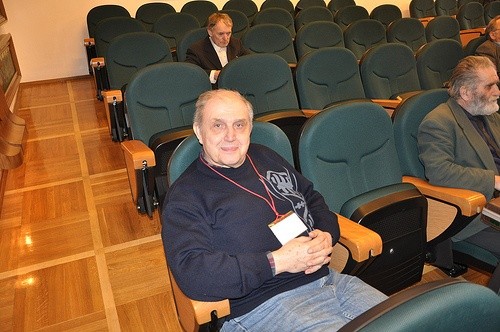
xmin=84 ymin=0 xmax=500 ymax=332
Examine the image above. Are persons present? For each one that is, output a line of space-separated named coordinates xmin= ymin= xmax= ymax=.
xmin=474 ymin=14 xmax=500 ymax=82
xmin=185 ymin=12 xmax=250 ymax=90
xmin=414 ymin=56 xmax=500 ymax=295
xmin=163 ymin=88 xmax=389 ymax=332
